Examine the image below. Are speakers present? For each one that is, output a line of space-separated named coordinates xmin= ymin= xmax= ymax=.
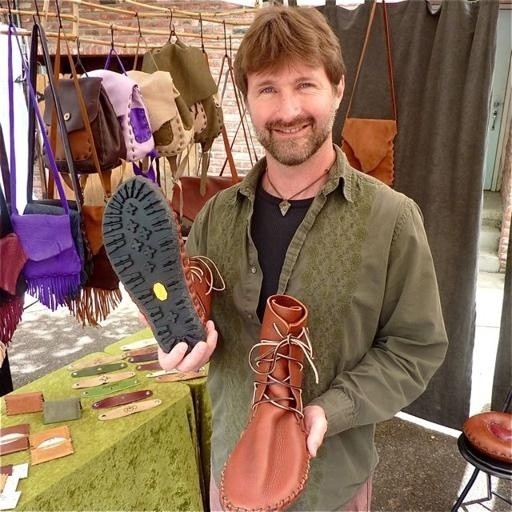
xmin=217 ymin=293 xmax=320 ymax=508
xmin=100 ymin=174 xmax=226 ymax=357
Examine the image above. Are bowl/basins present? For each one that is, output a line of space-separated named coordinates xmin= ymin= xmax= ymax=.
xmin=264 ymin=166 xmax=336 ymax=217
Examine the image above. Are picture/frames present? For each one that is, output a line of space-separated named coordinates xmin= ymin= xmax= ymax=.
xmin=439 ymin=386 xmax=512 ymax=512
xmin=104 ymin=326 xmax=214 ymax=482
xmin=2 ymin=355 xmax=204 ymax=510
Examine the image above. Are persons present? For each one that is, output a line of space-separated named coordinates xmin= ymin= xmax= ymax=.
xmin=138 ymin=5 xmax=449 ymax=512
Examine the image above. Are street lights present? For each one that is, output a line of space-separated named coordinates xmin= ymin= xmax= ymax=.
xmin=42 ymin=60 xmax=120 ymax=172
xmin=46 ymin=28 xmax=122 ymax=327
xmin=171 ymin=127 xmax=245 ymax=235
xmin=120 ymin=38 xmax=194 ymax=157
xmin=140 ymin=31 xmax=223 ymax=152
xmin=0 ymin=185 xmax=26 ymax=346
xmin=21 ymin=26 xmax=92 ymax=292
xmin=80 ymin=49 xmax=155 ymax=162
xmin=339 ymin=1 xmax=398 ymax=186
xmin=7 ymin=22 xmax=81 ymax=311
